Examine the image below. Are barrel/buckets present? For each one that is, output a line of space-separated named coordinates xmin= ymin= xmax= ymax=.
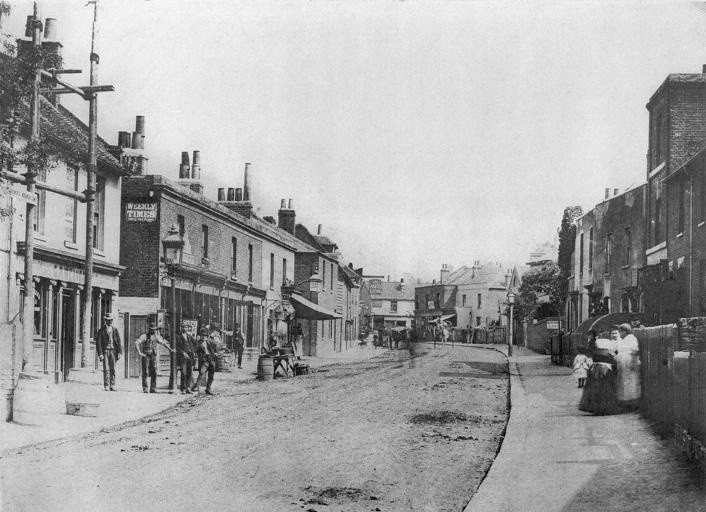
xmin=257 ymin=357 xmax=274 ymax=380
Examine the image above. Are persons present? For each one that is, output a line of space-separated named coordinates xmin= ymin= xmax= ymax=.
xmin=570 ymin=313 xmax=644 ymax=416
xmin=373 ymin=327 xmax=399 ymax=350
xmin=96 ymin=313 xmax=246 ymax=396
xmin=441 ymin=324 xmax=476 ymax=343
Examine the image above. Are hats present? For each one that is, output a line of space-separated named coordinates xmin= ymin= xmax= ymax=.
xmin=104 ymin=313 xmax=114 ymax=320
xmin=149 ymin=322 xmax=157 ymax=330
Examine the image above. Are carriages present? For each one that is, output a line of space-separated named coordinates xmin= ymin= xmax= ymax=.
xmin=427 ymin=319 xmax=456 ymax=349
